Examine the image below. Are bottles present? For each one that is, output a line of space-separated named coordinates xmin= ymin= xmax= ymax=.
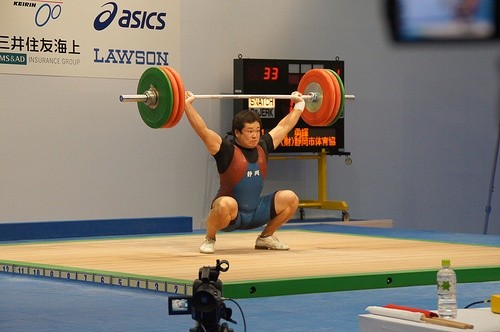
xmin=436 ymin=259 xmax=457 ymax=320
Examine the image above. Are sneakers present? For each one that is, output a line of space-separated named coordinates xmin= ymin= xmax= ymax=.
xmin=200 ymin=236 xmax=216 ymax=253
xmin=255 ymin=235 xmax=290 ymax=250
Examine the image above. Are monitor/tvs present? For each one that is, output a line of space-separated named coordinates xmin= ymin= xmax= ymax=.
xmin=387 ymin=0 xmax=500 ymax=43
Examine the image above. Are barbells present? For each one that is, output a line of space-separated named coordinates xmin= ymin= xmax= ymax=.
xmin=119 ymin=66 xmax=355 ymax=130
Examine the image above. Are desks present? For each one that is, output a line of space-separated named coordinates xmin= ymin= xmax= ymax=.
xmin=358 ymin=307 xmax=500 ymax=332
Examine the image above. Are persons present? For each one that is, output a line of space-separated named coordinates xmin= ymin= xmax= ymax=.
xmin=185 ymin=91 xmax=305 ymax=254
